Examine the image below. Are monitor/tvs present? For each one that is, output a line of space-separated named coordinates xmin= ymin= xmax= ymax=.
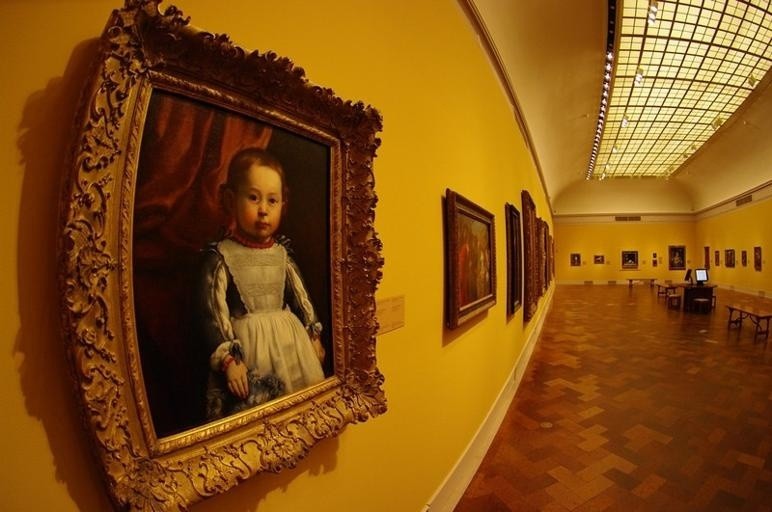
xmin=695 ymin=269 xmax=708 ymax=285
xmin=685 ymin=269 xmax=693 ymax=284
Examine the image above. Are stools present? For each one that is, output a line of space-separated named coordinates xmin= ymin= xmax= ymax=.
xmin=668 ymin=294 xmax=681 ymax=310
xmin=693 ymin=298 xmax=709 ymax=315
xmin=712 ymin=296 xmax=716 ymax=309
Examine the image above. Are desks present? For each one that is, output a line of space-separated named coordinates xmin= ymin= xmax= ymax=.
xmin=656 ymin=283 xmax=678 ymax=300
xmin=679 ymin=283 xmax=718 ymax=313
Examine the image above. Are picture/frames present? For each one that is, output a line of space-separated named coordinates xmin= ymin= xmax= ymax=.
xmin=570 ymin=245 xmax=686 ymax=271
xmin=704 ymin=246 xmax=761 ymax=270
xmin=59 ymin=0 xmax=387 ymax=512
xmin=445 ymin=188 xmax=497 ymax=331
xmin=505 ymin=190 xmax=555 ymax=322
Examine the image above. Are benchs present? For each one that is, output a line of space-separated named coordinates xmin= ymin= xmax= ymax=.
xmin=725 ymin=302 xmax=772 ymax=342
xmin=627 ymin=278 xmax=657 ymax=289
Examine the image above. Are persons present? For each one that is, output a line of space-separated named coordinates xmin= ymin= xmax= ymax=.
xmin=200 ymin=145 xmax=328 ymax=427
xmin=458 ymin=223 xmax=492 ymax=309
xmin=671 ymin=250 xmax=684 ymax=267
